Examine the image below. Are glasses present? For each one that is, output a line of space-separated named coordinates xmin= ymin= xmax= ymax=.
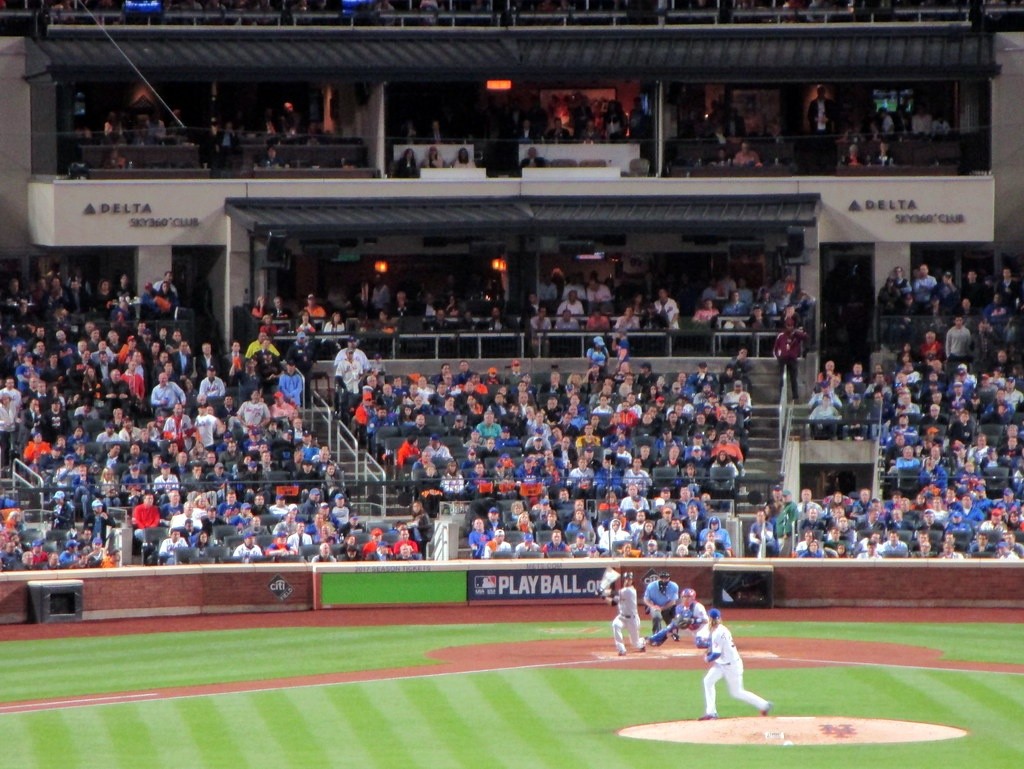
xmin=660 ymin=576 xmax=669 ymax=579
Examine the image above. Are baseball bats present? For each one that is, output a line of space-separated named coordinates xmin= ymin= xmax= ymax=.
xmin=595 ymin=566 xmax=621 ymax=595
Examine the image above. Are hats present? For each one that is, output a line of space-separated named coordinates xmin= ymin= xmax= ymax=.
xmin=659 ymin=571 xmax=671 ymax=580
xmin=623 ymin=571 xmax=634 ymax=579
xmin=708 ymin=608 xmax=720 ymax=619
xmin=0 ymin=251 xmax=1022 ymax=568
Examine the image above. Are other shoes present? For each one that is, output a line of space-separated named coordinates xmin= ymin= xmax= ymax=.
xmin=640 ymin=647 xmax=646 ymax=652
xmin=699 ymin=713 xmax=718 ymax=721
xmin=761 ymin=703 xmax=772 ymax=716
xmin=672 ymin=634 xmax=680 ymax=641
xmin=618 ymin=651 xmax=626 ymax=656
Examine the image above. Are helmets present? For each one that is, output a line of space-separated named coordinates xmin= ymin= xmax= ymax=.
xmin=681 ymin=588 xmax=696 ymax=598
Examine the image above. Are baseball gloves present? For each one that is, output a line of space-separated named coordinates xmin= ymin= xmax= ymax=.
xmin=675 ymin=615 xmax=691 ymax=628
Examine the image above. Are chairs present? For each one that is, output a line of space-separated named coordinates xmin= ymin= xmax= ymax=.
xmin=0 ymin=0 xmax=1024 ymax=566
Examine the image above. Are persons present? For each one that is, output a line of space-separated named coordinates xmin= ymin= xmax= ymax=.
xmin=600 ymin=572 xmax=647 ymax=657
xmin=675 ymin=588 xmax=711 ymax=648
xmin=694 ymin=608 xmax=770 ymax=722
xmin=69 ymin=80 xmax=958 ymax=171
xmin=641 ymin=572 xmax=681 ymax=642
xmin=2 ymin=251 xmax=1023 ymax=558
